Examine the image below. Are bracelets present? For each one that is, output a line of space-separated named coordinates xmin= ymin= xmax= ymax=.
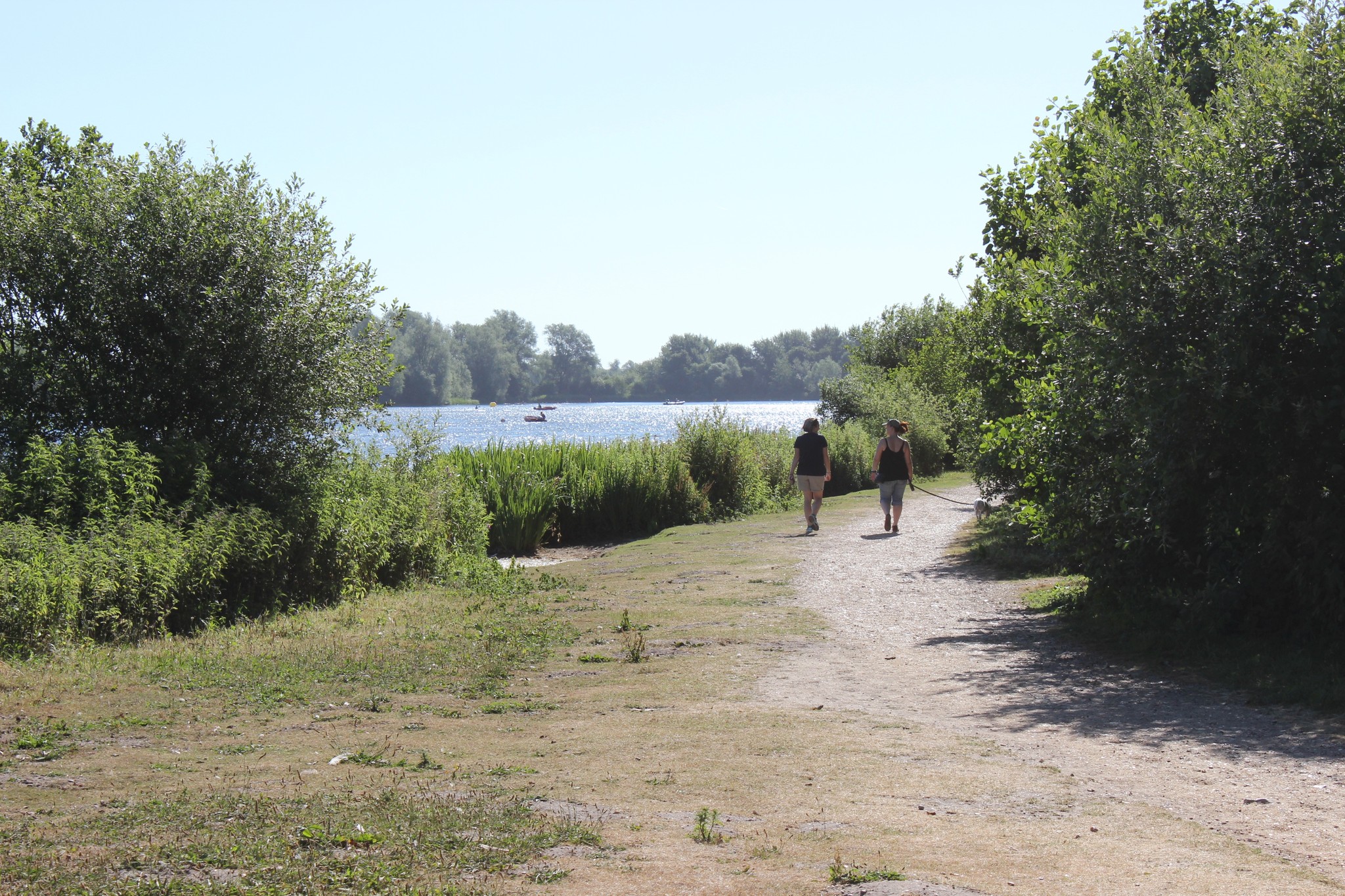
xmin=826 ymin=471 xmax=831 ymax=473
xmin=789 ymin=474 xmax=794 ymax=475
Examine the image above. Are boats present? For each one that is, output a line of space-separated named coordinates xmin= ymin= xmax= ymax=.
xmin=534 ymin=406 xmax=558 ymax=410
xmin=663 ymin=399 xmax=685 ymax=405
xmin=524 ymin=415 xmax=547 ymax=422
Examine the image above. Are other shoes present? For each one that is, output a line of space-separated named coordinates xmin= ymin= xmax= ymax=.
xmin=808 ymin=514 xmax=819 ymax=531
xmin=884 ymin=513 xmax=891 ymax=532
xmin=892 ymin=525 xmax=899 ymax=532
xmin=806 ymin=526 xmax=813 ymax=534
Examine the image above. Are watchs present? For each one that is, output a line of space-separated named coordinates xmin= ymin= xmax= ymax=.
xmin=871 ymin=470 xmax=877 ymax=473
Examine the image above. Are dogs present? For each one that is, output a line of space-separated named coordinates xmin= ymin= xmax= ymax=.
xmin=974 ymin=498 xmax=991 ymax=521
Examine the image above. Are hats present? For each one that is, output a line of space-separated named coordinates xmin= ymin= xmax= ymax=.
xmin=882 ymin=418 xmax=900 ymax=428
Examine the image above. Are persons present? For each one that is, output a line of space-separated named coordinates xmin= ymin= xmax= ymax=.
xmin=789 ymin=417 xmax=832 ymax=533
xmin=871 ymin=419 xmax=913 ymax=533
